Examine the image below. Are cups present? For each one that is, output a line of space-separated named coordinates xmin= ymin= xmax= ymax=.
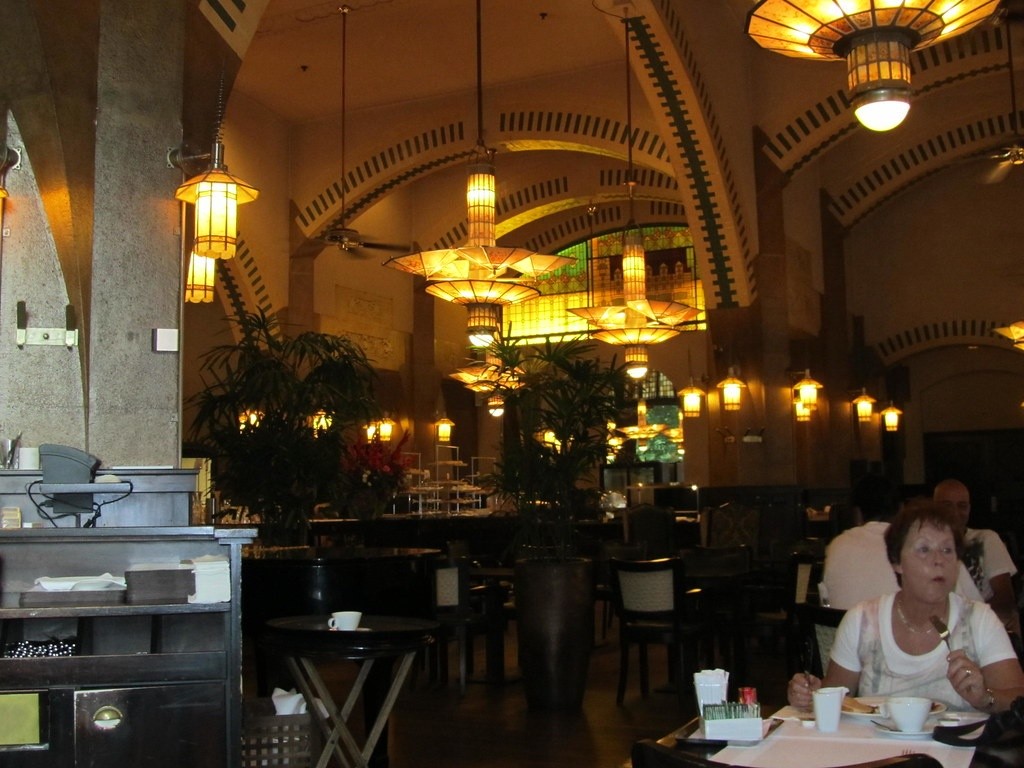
xmin=328 ymin=611 xmax=362 ymax=631
xmin=813 ymin=686 xmax=850 ymax=733
xmin=888 ymin=697 xmax=933 ymax=734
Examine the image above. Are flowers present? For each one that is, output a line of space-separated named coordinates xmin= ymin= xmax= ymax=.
xmin=328 ymin=420 xmax=418 ymax=491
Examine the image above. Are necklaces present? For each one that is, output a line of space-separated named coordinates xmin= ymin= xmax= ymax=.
xmin=897 ymin=597 xmax=945 ymax=634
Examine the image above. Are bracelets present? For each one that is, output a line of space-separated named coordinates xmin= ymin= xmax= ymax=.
xmin=984 ymin=688 xmax=996 ymax=710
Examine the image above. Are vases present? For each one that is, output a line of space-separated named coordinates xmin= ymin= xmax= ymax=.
xmin=342 ymin=474 xmax=397 ymax=523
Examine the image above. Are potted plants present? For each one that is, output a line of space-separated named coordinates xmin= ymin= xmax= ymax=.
xmin=490 ymin=339 xmax=642 ymax=717
xmin=177 ymin=303 xmax=411 ymax=549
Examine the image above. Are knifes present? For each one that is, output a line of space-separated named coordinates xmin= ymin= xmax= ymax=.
xmin=800 ymin=635 xmax=812 ymax=679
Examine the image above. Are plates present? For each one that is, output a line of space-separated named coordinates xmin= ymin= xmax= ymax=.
xmin=840 ymin=696 xmax=947 ymax=723
xmin=875 ymin=721 xmax=934 ymax=739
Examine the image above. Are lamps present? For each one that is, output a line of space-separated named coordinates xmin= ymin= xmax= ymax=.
xmin=564 ymin=0 xmax=705 ymax=380
xmin=237 ymin=407 xmax=455 ymax=447
xmin=447 ymin=303 xmax=528 ymax=418
xmin=164 ymin=142 xmax=260 ymax=305
xmin=744 ymin=0 xmax=1002 ymax=132
xmin=535 ymin=366 xmax=903 ymax=464
xmin=382 ymin=0 xmax=579 ymax=350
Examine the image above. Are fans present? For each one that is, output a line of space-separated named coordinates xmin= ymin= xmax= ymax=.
xmin=942 ymin=14 xmax=1024 ymax=186
xmin=285 ymin=6 xmax=413 ymax=263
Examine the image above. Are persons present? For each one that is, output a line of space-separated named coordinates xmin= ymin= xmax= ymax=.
xmin=934 ymin=479 xmax=1017 ymax=612
xmin=787 ymin=497 xmax=1024 ymax=713
xmin=816 ymin=499 xmax=901 ymax=678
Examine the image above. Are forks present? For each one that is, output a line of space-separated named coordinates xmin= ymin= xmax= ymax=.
xmin=929 ymin=614 xmax=952 ymax=653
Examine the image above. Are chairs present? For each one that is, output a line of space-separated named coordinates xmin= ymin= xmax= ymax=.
xmin=583 ymin=484 xmax=1024 ymax=707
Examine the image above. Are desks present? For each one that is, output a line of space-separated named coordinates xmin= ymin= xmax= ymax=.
xmin=308 ymin=510 xmax=702 ymax=703
xmin=265 ymin=614 xmax=441 ymax=768
xmin=468 ymin=565 xmax=516 ymax=689
xmin=629 ymin=703 xmax=1024 ymax=768
xmin=239 ymin=544 xmax=441 ymax=702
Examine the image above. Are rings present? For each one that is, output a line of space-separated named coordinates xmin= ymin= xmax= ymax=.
xmin=966 ymin=667 xmax=972 ymax=677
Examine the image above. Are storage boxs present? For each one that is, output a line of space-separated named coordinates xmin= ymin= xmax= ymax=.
xmin=697 ymin=714 xmax=763 ymax=742
xmin=124 ymin=553 xmax=232 ymax=606
